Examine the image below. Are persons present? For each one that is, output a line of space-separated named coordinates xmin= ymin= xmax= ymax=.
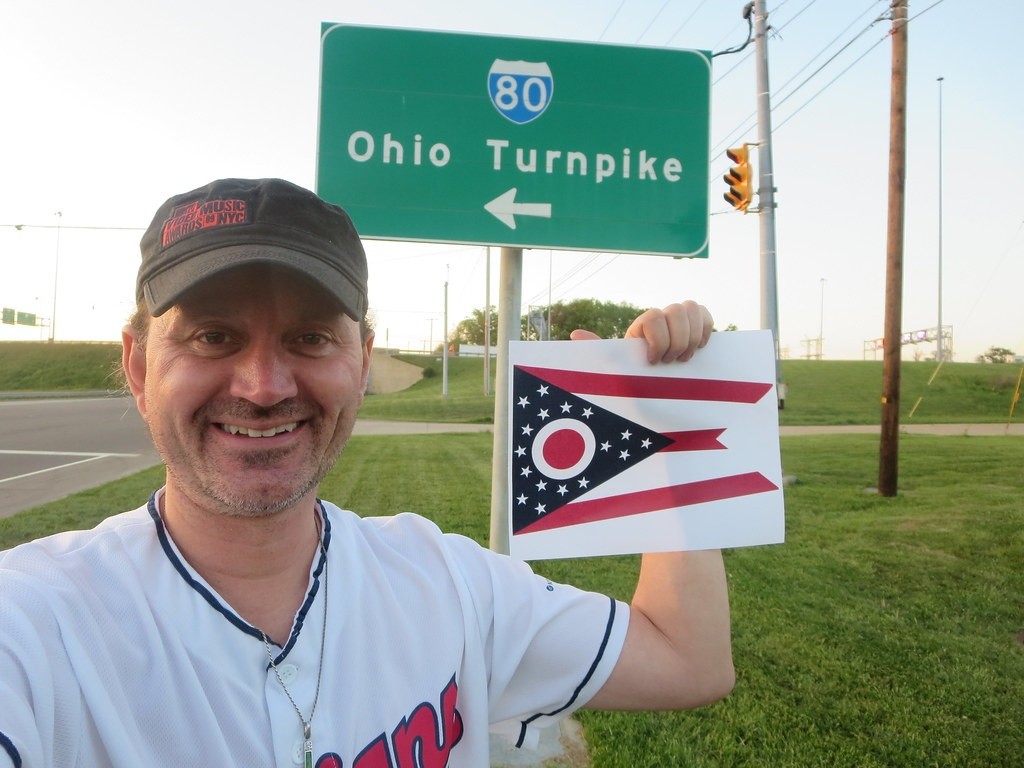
xmin=0 ymin=178 xmax=736 ymax=768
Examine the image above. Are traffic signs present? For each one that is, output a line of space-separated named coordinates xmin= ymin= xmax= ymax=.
xmin=722 ymin=142 xmax=753 ymax=209
xmin=311 ymin=22 xmax=711 ymax=260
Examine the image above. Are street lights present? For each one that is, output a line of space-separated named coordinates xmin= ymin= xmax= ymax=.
xmin=937 ymin=76 xmax=950 ymax=367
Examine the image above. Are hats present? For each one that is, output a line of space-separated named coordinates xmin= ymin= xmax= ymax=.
xmin=136 ymin=178 xmax=369 ymax=321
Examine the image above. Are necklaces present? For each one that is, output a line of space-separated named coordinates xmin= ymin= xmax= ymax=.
xmin=253 ymin=514 xmax=328 ymax=768
